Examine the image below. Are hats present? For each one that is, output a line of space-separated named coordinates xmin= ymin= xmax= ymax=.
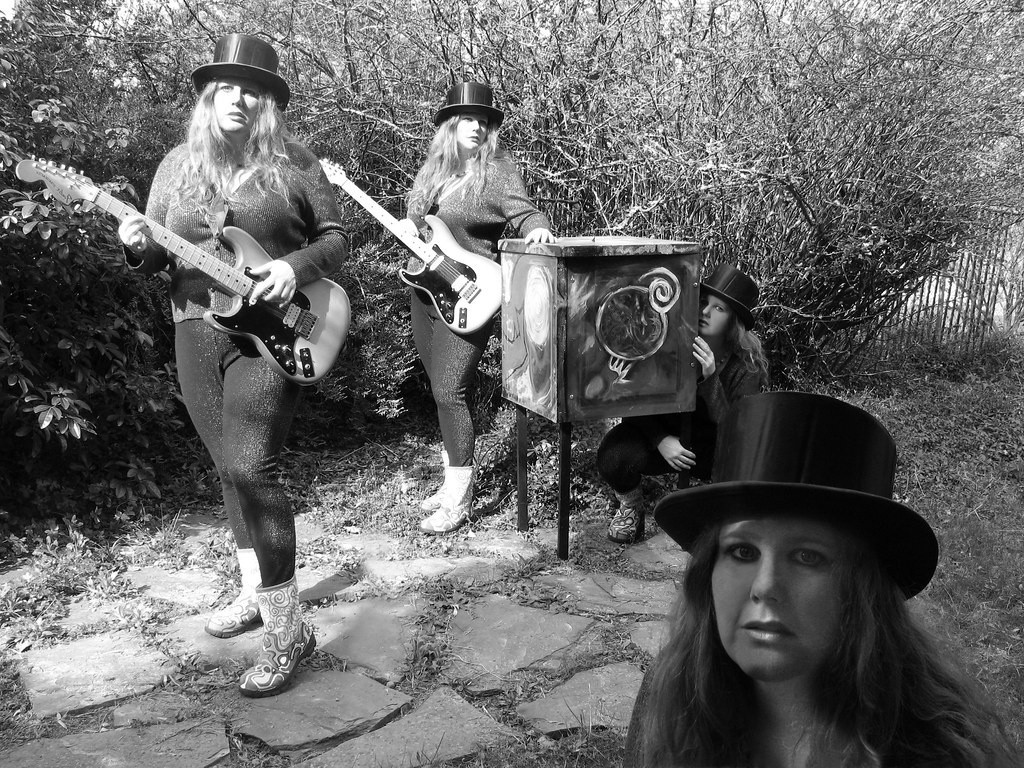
xmin=434 ymin=82 xmax=504 ymax=127
xmin=701 ymin=263 xmax=760 ymax=330
xmin=190 ymin=34 xmax=290 ymax=112
xmin=652 ymin=390 xmax=938 ymax=601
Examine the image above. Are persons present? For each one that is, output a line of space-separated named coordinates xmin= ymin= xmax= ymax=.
xmin=596 ymin=264 xmax=764 ymax=543
xmin=397 ymin=81 xmax=555 ymax=533
xmin=118 ymin=33 xmax=350 ymax=696
xmin=619 ymin=393 xmax=1024 ymax=767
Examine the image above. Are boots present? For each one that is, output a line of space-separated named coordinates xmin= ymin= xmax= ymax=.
xmin=608 ymin=481 xmax=647 ymax=542
xmin=234 ymin=575 xmax=319 ymax=698
xmin=421 ymin=464 xmax=477 ymax=533
xmin=207 ymin=548 xmax=262 ymax=638
xmin=420 ymin=450 xmax=450 ymax=513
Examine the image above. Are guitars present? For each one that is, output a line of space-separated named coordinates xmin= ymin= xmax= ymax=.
xmin=14 ymin=153 xmax=353 ymax=388
xmin=314 ymin=156 xmax=505 ymax=336
